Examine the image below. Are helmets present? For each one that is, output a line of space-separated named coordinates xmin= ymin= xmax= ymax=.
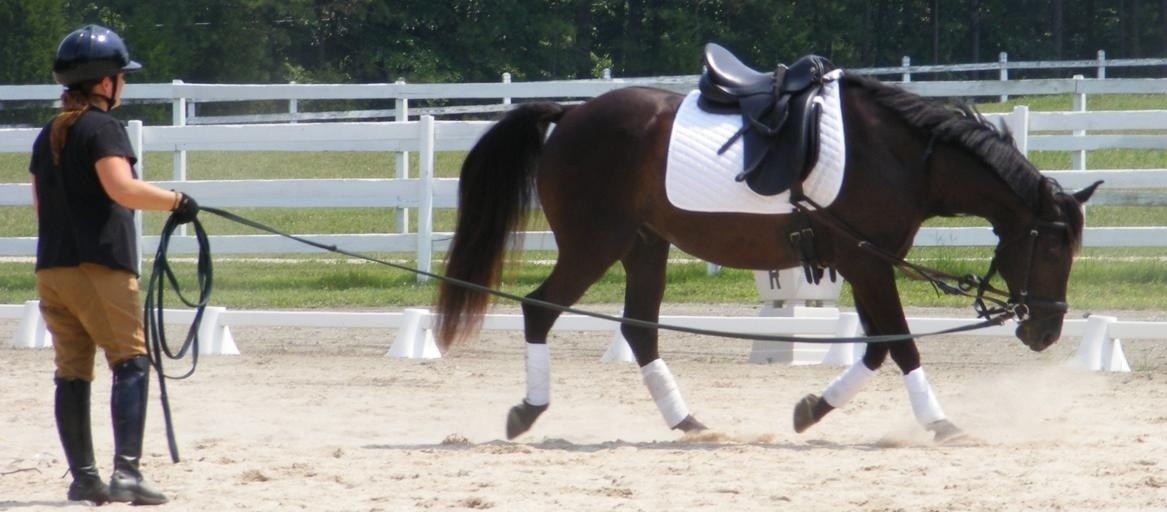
xmin=52 ymin=24 xmax=143 ymax=86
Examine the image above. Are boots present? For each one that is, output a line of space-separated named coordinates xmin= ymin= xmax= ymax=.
xmin=108 ymin=354 xmax=169 ymax=504
xmin=54 ymin=376 xmax=110 ymax=502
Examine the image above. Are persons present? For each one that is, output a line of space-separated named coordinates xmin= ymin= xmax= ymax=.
xmin=26 ymin=24 xmax=200 ymax=505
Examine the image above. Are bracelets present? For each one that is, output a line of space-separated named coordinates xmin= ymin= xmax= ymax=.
xmin=170 ymin=187 xmax=180 ymax=212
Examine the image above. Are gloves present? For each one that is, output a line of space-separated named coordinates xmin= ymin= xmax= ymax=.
xmin=171 ymin=190 xmax=200 ymax=225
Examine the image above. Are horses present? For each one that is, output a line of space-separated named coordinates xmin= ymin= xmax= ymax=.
xmin=429 ymin=71 xmax=1106 ymax=446
